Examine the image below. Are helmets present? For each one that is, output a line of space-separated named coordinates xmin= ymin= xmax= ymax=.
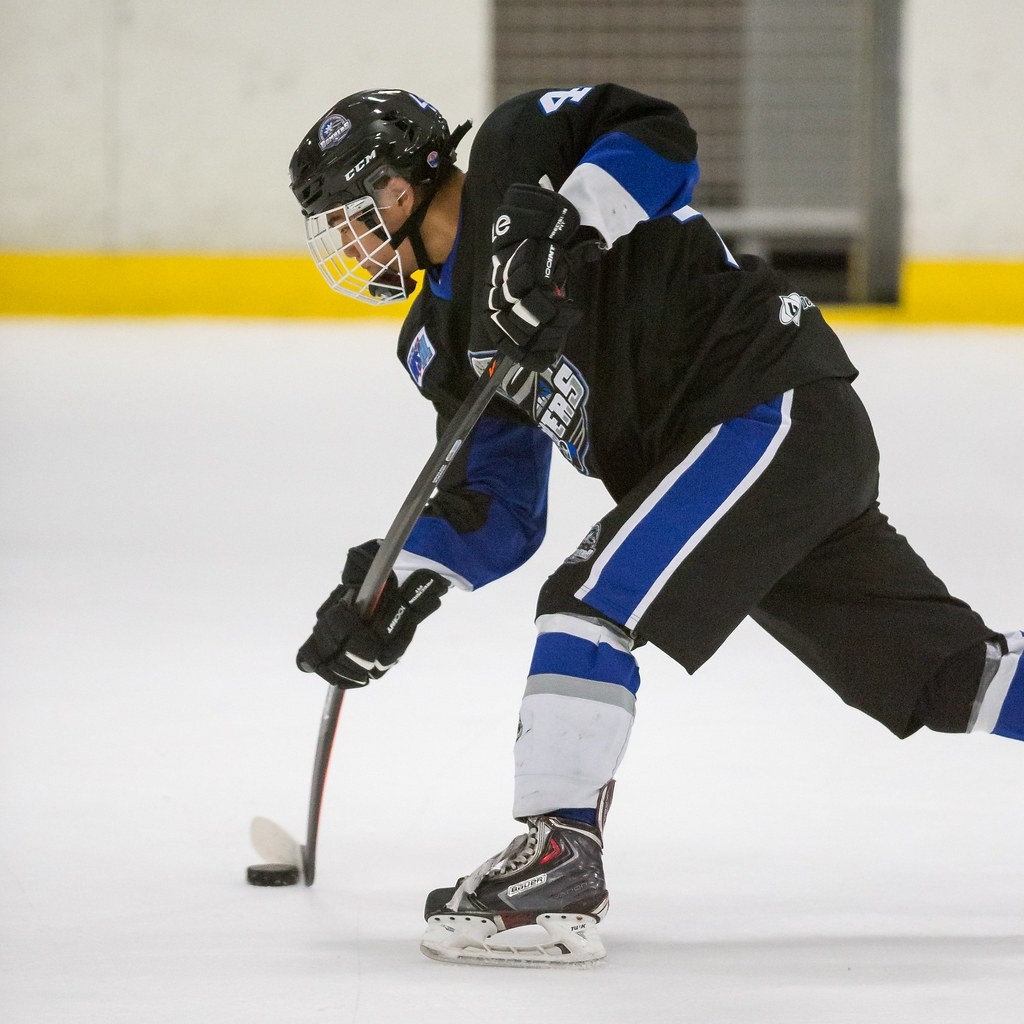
xmin=287 ymin=87 xmax=453 ymax=220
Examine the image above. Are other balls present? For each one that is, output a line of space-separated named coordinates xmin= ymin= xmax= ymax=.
xmin=245 ymin=864 xmax=300 ymax=888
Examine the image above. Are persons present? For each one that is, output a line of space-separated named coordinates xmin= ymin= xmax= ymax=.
xmin=286 ymin=81 xmax=1024 ymax=969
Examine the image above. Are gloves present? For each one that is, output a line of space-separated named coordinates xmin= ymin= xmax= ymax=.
xmin=480 ymin=184 xmax=605 ymax=371
xmin=295 ymin=540 xmax=451 ymax=691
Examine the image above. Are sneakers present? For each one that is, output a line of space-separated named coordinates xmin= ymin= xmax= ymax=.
xmin=419 ymin=813 xmax=611 ymax=968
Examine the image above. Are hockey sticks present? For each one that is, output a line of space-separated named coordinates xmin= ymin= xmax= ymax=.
xmin=244 ymin=269 xmax=571 ymax=891
